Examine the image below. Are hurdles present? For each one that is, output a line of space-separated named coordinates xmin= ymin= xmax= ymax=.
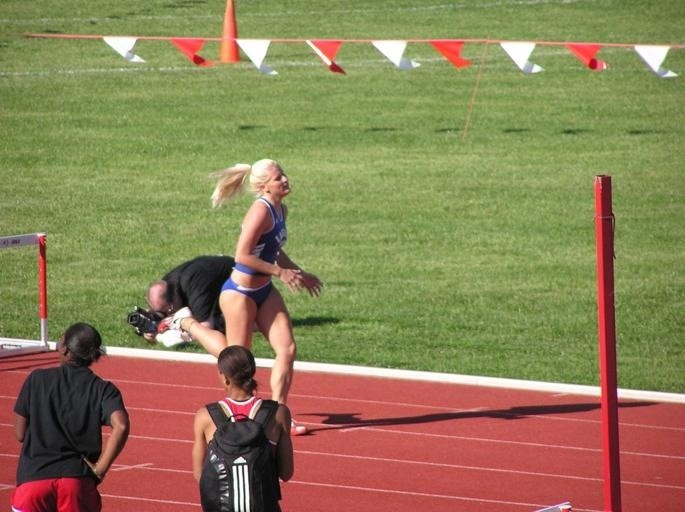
xmin=0 ymin=233 xmax=57 ymax=359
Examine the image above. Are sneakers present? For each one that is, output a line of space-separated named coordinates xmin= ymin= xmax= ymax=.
xmin=290 ymin=419 xmax=307 ymax=437
xmin=156 ymin=306 xmax=194 ymax=335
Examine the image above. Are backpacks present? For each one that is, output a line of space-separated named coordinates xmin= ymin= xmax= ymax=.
xmin=199 ymin=400 xmax=282 ymax=512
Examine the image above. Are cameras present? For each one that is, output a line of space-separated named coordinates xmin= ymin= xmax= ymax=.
xmin=128 ymin=306 xmax=167 ymax=334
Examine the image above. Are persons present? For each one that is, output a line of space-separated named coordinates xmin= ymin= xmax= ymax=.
xmin=192 ymin=346 xmax=293 ymax=512
xmin=157 ymin=159 xmax=324 ymax=435
xmin=143 ymin=256 xmax=235 ymax=343
xmin=11 ymin=323 xmax=130 ymax=512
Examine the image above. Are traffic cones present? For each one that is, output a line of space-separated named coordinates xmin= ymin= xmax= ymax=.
xmin=220 ymin=0 xmax=240 ymax=64
